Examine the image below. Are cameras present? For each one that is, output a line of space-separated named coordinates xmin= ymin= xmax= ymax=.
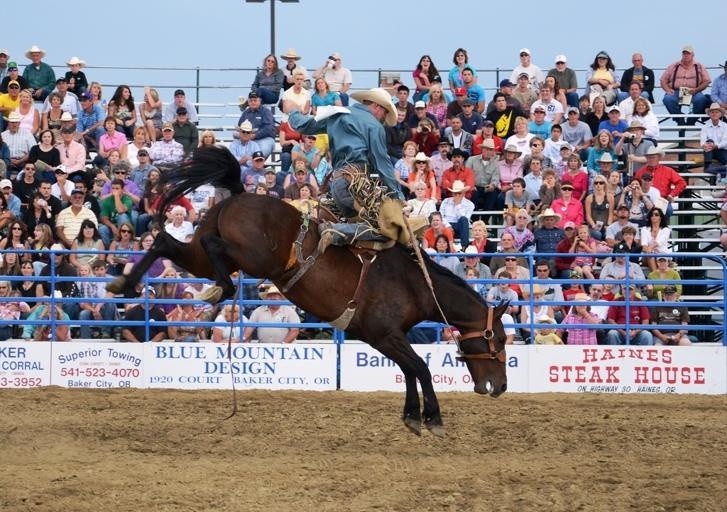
xmin=422 ymin=127 xmax=429 ymax=132
xmin=628 ymin=134 xmax=637 ymax=139
xmin=630 ymin=186 xmax=636 ymax=190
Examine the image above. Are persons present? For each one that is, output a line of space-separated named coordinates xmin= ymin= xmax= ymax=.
xmin=281 ymin=46 xmax=726 ymax=345
xmin=282 ymin=87 xmax=406 ymax=254
xmin=1 ymin=46 xmax=300 ymax=345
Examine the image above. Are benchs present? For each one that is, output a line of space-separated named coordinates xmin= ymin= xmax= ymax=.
xmin=70 ymin=100 xmax=727 ymax=345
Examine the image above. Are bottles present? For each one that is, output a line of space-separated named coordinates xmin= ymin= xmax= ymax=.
xmin=715 ymin=172 xmax=721 ymax=186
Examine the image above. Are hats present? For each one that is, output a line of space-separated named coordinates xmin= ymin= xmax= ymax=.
xmin=350 ymin=88 xmax=398 ymax=127
xmin=706 ymin=101 xmax=725 ymax=117
xmin=0 ymin=45 xmax=307 ymax=299
xmin=683 ymin=44 xmax=692 ymax=54
xmin=414 ymin=47 xmax=676 ymax=333
xmin=328 ymin=52 xmax=342 ymax=62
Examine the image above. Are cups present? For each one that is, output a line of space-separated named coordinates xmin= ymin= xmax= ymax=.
xmin=327 ymin=60 xmax=336 ymax=69
xmin=595 ymin=221 xmax=603 ymax=232
xmin=37 ymin=199 xmax=47 ymax=207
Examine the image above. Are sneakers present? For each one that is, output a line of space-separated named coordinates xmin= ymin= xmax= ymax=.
xmin=317 ymin=220 xmax=333 ymax=256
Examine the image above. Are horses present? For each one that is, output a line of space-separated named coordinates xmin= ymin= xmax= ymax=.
xmin=104 ymin=143 xmax=511 ymax=438
